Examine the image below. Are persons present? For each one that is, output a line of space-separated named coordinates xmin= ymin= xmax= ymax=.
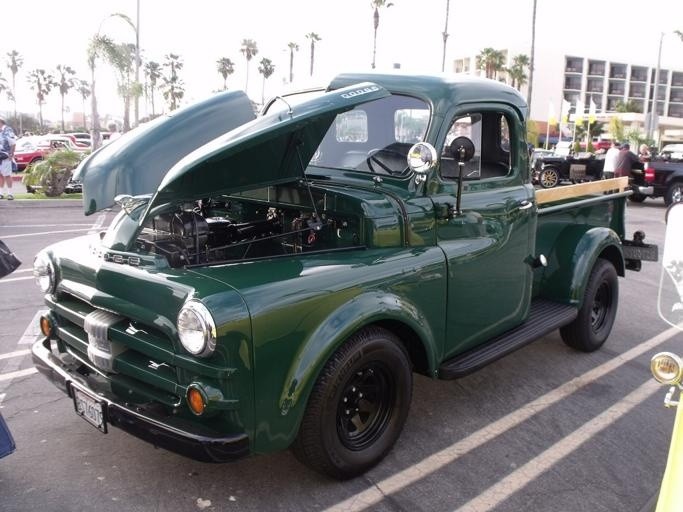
xmin=0 ymin=114 xmax=17 ymax=200
xmin=602 ymin=142 xmax=621 ymax=195
xmin=613 ymin=143 xmax=641 ymax=193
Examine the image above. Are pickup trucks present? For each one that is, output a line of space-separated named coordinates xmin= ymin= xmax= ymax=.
xmin=628 ymin=143 xmax=682 ymax=206
xmin=29 ymin=72 xmax=660 ymax=484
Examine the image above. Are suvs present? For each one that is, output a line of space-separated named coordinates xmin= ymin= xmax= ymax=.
xmin=530 ymin=141 xmax=604 ymax=190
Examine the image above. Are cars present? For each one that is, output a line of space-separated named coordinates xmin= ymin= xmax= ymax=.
xmin=11 ymin=131 xmax=114 ymax=193
